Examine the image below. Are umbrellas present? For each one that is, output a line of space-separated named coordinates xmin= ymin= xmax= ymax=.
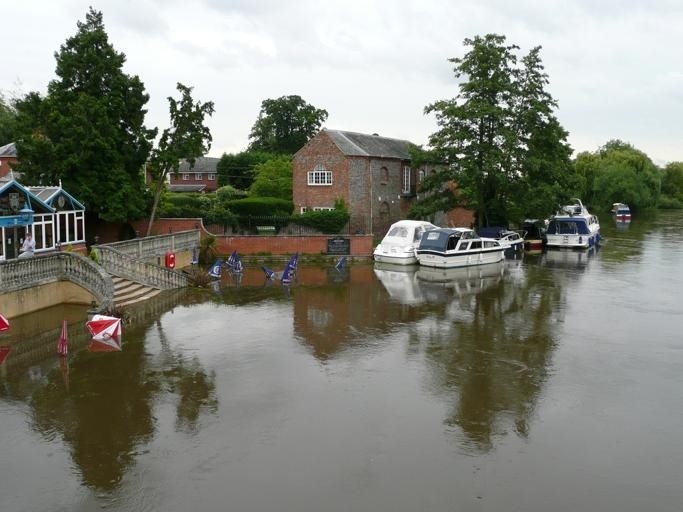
xmin=0 ymin=312 xmax=10 ymax=332
xmin=57 ymin=317 xmax=68 ymax=356
xmin=86 ymin=313 xmax=123 ymax=352
xmin=189 ymin=244 xmax=347 ymax=284
xmin=59 ymin=354 xmax=71 ymax=391
xmin=0 ymin=345 xmax=11 ymax=366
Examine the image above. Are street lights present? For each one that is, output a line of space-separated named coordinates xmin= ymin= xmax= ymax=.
xmin=18 ymin=201 xmax=33 ymax=238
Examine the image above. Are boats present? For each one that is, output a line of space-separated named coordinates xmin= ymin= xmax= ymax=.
xmin=370 ymin=262 xmax=508 ymax=306
xmin=370 ymin=195 xmax=632 ymax=268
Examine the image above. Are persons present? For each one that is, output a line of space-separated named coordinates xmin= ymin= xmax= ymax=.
xmin=17 ymin=232 xmax=36 ymax=258
